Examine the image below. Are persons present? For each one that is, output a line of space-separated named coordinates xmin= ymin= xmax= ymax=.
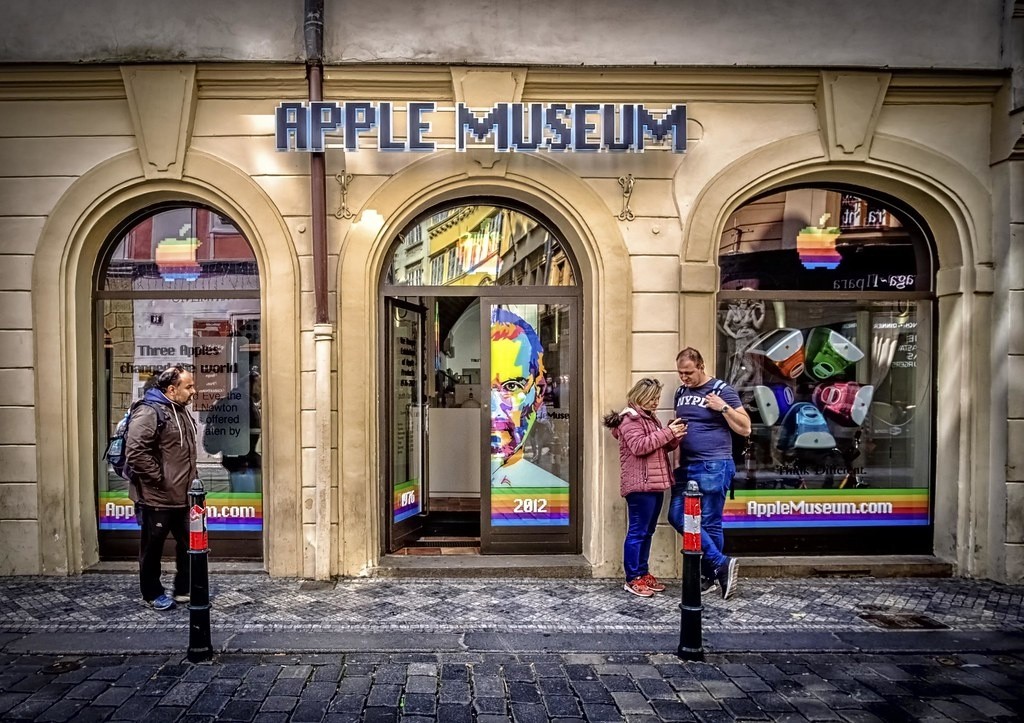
xmin=667 ymin=346 xmax=751 ymax=599
xmin=601 ymin=378 xmax=688 ymax=597
xmin=122 ymin=366 xmax=198 ymax=611
xmin=220 ymin=369 xmax=261 ymax=550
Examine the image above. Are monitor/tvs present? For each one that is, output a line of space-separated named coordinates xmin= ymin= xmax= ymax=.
xmin=736 ymin=327 xmax=876 ymax=448
xmin=454 ymin=385 xmax=481 ymax=408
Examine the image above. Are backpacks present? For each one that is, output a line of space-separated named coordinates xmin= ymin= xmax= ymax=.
xmin=202 ymin=391 xmax=251 ymax=454
xmin=102 ymin=399 xmax=167 ymax=480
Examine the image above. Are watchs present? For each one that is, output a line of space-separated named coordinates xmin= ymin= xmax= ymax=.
xmin=720 ymin=404 xmax=729 ymax=414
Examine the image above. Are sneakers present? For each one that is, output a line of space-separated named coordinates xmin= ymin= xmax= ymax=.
xmin=624 ymin=575 xmax=655 ymax=597
xmin=640 ymin=573 xmax=666 ymax=592
xmin=701 ymin=573 xmax=721 ymax=596
xmin=145 ymin=593 xmax=176 ymax=611
xmin=173 ymin=592 xmax=190 ymax=603
xmin=713 ymin=557 xmax=740 ymax=599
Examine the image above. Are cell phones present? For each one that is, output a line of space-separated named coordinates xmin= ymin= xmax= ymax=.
xmin=668 ymin=419 xmax=688 ymax=426
